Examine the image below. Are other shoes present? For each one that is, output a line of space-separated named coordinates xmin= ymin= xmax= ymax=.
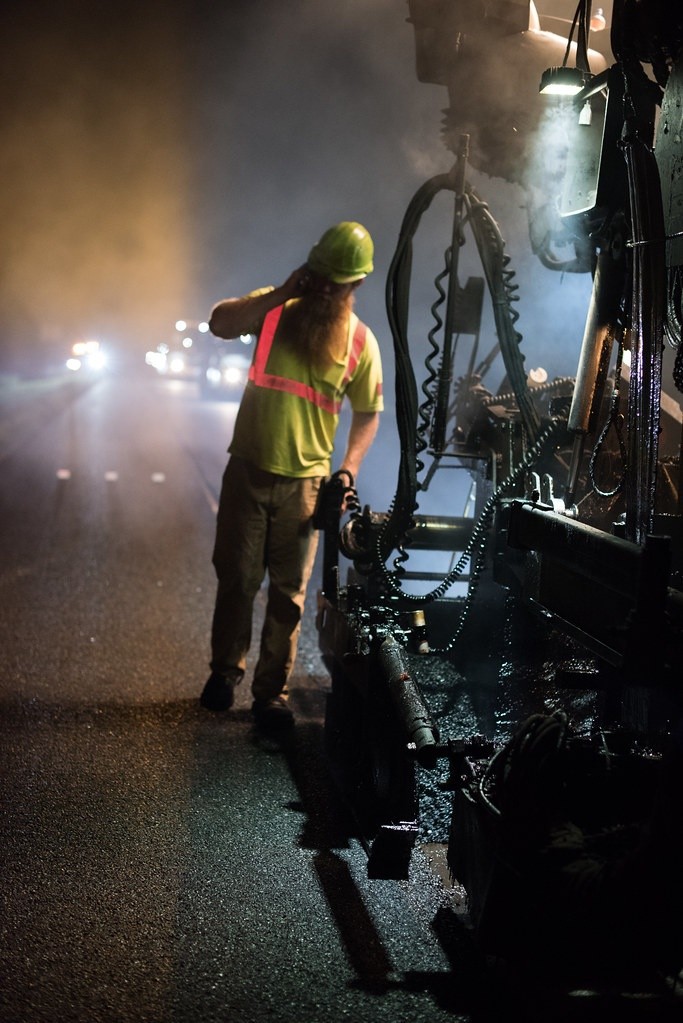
xmin=251 ymin=696 xmax=293 ymax=724
xmin=199 ymin=668 xmax=236 ymax=711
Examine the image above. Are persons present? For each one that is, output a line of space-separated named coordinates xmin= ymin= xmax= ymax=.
xmin=202 ymin=222 xmax=384 ymax=723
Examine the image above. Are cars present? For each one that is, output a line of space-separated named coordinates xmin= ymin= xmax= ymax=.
xmin=150 ymin=322 xmax=258 ymax=395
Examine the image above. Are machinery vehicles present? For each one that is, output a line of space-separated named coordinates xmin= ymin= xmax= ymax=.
xmin=312 ymin=1 xmax=683 ymax=1023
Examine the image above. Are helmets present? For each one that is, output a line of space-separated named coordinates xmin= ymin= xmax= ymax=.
xmin=307 ymin=221 xmax=374 ymax=284
